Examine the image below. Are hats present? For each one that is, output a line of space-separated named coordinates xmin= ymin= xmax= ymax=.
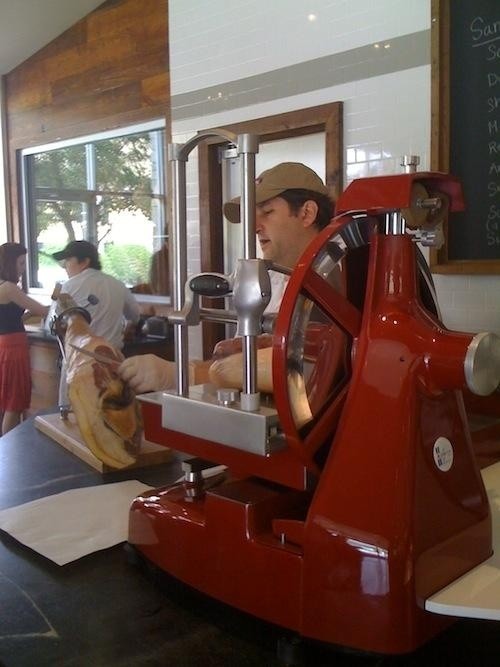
xmin=52 ymin=241 xmax=99 ymax=259
xmin=224 ymin=162 xmax=330 ymax=223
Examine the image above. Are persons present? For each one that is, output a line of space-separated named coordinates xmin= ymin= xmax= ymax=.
xmin=115 ymin=161 xmax=333 ymax=395
xmin=45 ymin=239 xmax=143 ymax=412
xmin=0 ymin=240 xmax=53 ymax=437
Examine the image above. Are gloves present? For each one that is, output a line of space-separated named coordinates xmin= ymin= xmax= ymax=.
xmin=116 ymin=353 xmax=196 ymax=393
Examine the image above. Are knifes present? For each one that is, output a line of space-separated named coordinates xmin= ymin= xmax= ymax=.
xmin=69 ymin=343 xmax=123 ymax=365
